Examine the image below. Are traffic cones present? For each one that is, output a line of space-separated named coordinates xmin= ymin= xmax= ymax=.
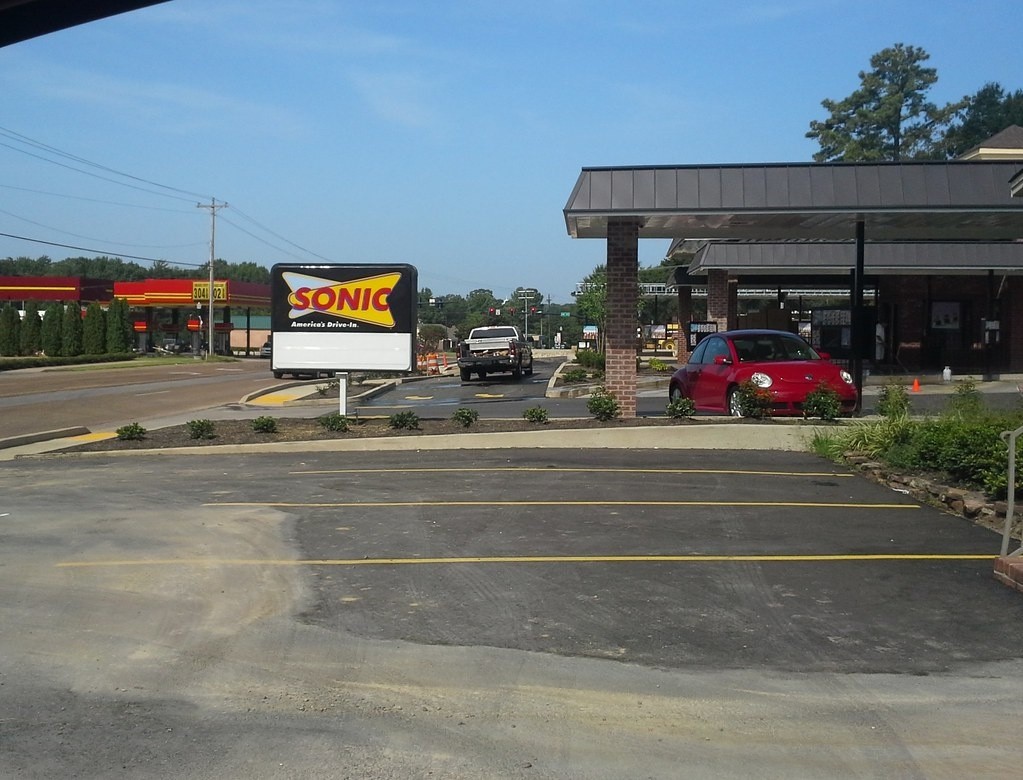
xmin=911 ymin=378 xmax=922 ymax=393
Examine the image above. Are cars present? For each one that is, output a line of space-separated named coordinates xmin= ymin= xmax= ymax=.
xmin=270 ymin=359 xmax=336 ymax=380
xmin=260 ymin=342 xmax=271 ymax=357
xmin=667 ymin=329 xmax=859 ymax=419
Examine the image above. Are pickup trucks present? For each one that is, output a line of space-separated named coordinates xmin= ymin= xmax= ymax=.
xmin=455 ymin=324 xmax=533 ymax=381
